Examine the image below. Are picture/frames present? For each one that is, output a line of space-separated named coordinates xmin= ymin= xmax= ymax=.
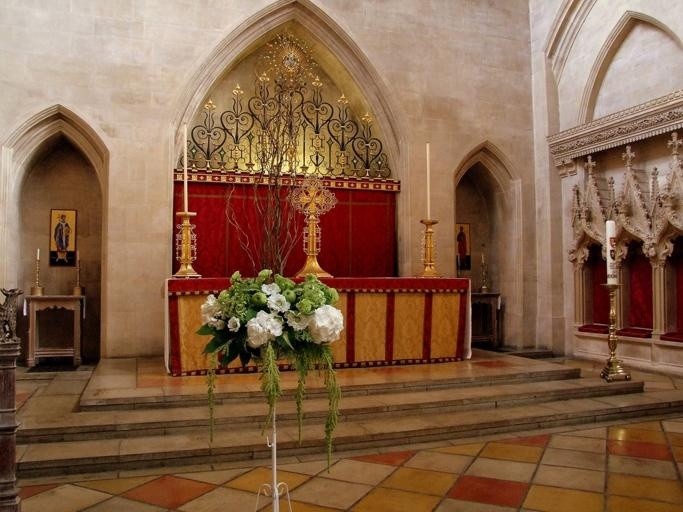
xmin=453 ymin=221 xmax=472 ymax=271
xmin=48 ymin=207 xmax=78 ymax=268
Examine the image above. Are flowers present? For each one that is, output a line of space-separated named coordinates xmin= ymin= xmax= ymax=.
xmin=200 ymin=265 xmax=344 ymax=477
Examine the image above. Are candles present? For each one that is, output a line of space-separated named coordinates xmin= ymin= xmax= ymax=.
xmin=606 ymin=219 xmax=621 ymax=284
xmin=425 ymin=141 xmax=431 ymax=218
xmin=36 ymin=248 xmax=40 ymax=260
xmin=77 ymin=250 xmax=80 ymax=260
xmin=182 ymin=128 xmax=189 ymax=210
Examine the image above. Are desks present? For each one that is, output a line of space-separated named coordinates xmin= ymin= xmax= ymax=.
xmin=471 ymin=290 xmax=502 ymax=346
xmin=24 ymin=292 xmax=85 ymax=373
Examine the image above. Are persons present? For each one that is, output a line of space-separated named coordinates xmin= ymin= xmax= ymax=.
xmin=457 ymin=226 xmax=466 ymax=263
xmin=54 ymin=215 xmax=71 ymax=250
